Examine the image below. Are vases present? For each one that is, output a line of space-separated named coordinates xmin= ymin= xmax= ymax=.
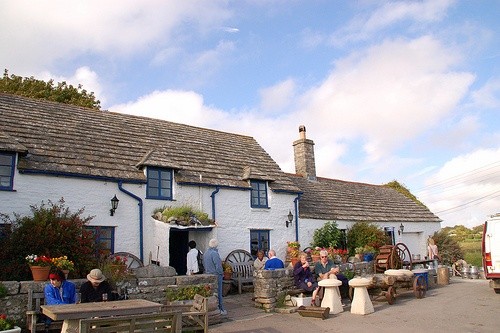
xmin=167 ymin=296 xmax=218 ymax=312
xmin=110 ymin=264 xmax=127 ymax=276
xmin=30 ymin=266 xmax=69 ymax=281
xmin=0 ymin=325 xmax=21 ymax=333
xmin=290 ymin=257 xmax=298 ymax=266
xmin=311 ymin=255 xmax=320 ymax=262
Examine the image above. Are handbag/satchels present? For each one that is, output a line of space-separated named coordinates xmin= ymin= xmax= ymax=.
xmin=433 ymin=254 xmax=442 ymax=263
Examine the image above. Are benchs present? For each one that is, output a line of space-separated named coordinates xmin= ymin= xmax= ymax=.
xmin=286 ymin=288 xmax=306 ymax=295
xmin=26 ymin=287 xmax=120 ymax=333
xmin=230 ymin=262 xmax=255 ymax=293
xmin=79 ymin=310 xmax=182 ymax=333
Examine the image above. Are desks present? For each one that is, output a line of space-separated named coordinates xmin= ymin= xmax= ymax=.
xmin=400 ymin=260 xmax=435 ymax=269
xmin=40 ymin=298 xmax=163 ymax=333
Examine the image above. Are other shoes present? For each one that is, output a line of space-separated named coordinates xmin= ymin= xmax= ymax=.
xmin=220 ymin=307 xmax=227 ymax=315
xmin=341 ymin=299 xmax=345 ymax=306
xmin=311 ymin=298 xmax=315 ymax=307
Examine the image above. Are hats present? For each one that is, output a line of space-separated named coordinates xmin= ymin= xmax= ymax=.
xmin=87 ymin=268 xmax=106 ymax=283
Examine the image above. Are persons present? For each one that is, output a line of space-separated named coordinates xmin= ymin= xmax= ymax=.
xmin=202 ymin=239 xmax=227 ymax=316
xmin=427 ymin=237 xmax=439 ymax=278
xmin=185 ymin=240 xmax=203 ymax=276
xmin=45 ymin=265 xmax=76 ymax=326
xmin=253 ymin=248 xmax=284 ymax=270
xmin=78 ymin=269 xmax=121 ymax=303
xmin=292 ymin=249 xmax=352 ymax=307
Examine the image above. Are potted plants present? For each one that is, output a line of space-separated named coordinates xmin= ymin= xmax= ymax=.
xmin=224 ymin=265 xmax=233 ymax=279
xmin=355 ymin=244 xmax=379 ymax=261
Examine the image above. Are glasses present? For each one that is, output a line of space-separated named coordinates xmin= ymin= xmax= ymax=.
xmin=321 ymin=256 xmax=327 ymax=258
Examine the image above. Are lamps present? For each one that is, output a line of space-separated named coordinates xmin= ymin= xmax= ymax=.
xmin=286 ymin=211 xmax=293 ymax=228
xmin=398 ymin=223 xmax=404 ymax=235
xmin=109 ymin=194 xmax=119 ymax=216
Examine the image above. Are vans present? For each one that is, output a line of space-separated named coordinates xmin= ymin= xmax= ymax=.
xmin=482 ymin=213 xmax=500 ymax=295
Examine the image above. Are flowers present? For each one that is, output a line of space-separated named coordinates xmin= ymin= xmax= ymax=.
xmin=166 ymin=283 xmax=212 ymax=301
xmin=25 ymin=254 xmax=75 ymax=271
xmin=0 ymin=313 xmax=16 ymax=331
xmin=106 ymin=254 xmax=127 ymax=264
xmin=286 ymin=241 xmax=348 ymax=257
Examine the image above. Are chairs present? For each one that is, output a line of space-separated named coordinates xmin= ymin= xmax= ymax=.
xmin=181 ymin=294 xmax=209 ymax=333
